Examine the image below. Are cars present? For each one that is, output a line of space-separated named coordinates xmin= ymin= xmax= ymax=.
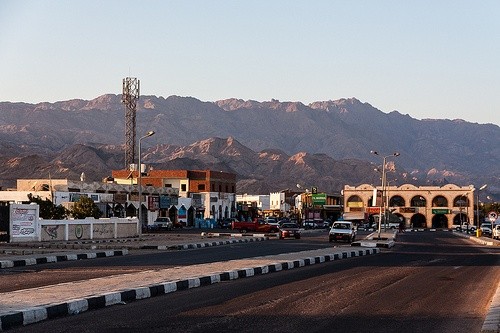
xmin=278 ymin=223 xmax=301 ymax=240
xmin=142 ymin=221 xmax=184 ymax=234
xmin=358 ymin=221 xmax=400 ymax=231
xmin=214 ymin=214 xmax=332 ymax=237
xmin=154 ymin=217 xmax=174 ymax=232
xmin=492 ymin=224 xmax=500 ymax=239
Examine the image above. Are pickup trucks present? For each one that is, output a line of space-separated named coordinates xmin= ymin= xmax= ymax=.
xmin=450 ymin=221 xmax=477 ymax=231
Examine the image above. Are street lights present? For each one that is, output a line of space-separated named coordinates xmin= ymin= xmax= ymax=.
xmin=476 ymin=184 xmax=488 ymax=229
xmin=370 ymin=148 xmax=400 ymax=238
xmin=138 ymin=130 xmax=155 ymax=234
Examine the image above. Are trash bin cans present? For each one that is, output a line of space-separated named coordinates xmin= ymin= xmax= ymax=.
xmin=477 ymin=229 xmax=481 ymax=237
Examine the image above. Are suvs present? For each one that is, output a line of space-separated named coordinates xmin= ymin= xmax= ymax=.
xmin=328 ymin=220 xmax=355 ymax=245
xmin=480 ymin=222 xmax=495 ymax=237
